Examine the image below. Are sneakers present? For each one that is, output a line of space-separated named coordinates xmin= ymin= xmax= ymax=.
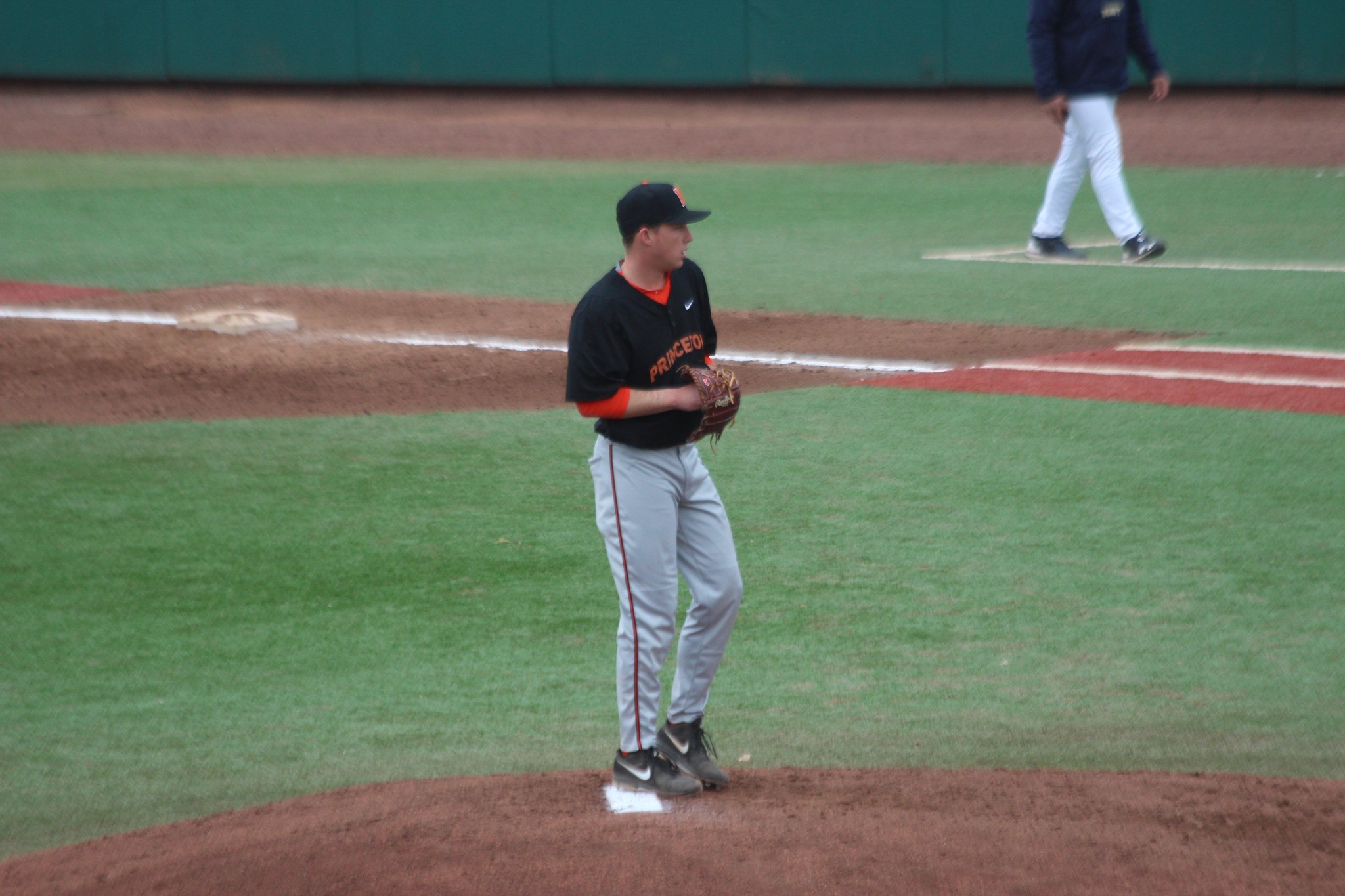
xmin=653 ymin=716 xmax=730 ymax=788
xmin=610 ymin=744 xmax=703 ymax=797
xmin=1120 ymin=233 xmax=1166 ymax=264
xmin=1024 ymin=235 xmax=1089 ymax=264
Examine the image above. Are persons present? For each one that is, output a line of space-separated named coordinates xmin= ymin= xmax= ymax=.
xmin=1022 ymin=0 xmax=1172 ymax=263
xmin=566 ymin=180 xmax=744 ymax=797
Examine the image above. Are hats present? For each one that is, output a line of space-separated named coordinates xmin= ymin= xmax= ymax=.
xmin=616 ymin=182 xmax=712 ymax=238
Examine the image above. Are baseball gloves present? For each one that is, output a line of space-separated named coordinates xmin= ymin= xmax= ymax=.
xmin=682 ymin=362 xmax=742 ymax=444
